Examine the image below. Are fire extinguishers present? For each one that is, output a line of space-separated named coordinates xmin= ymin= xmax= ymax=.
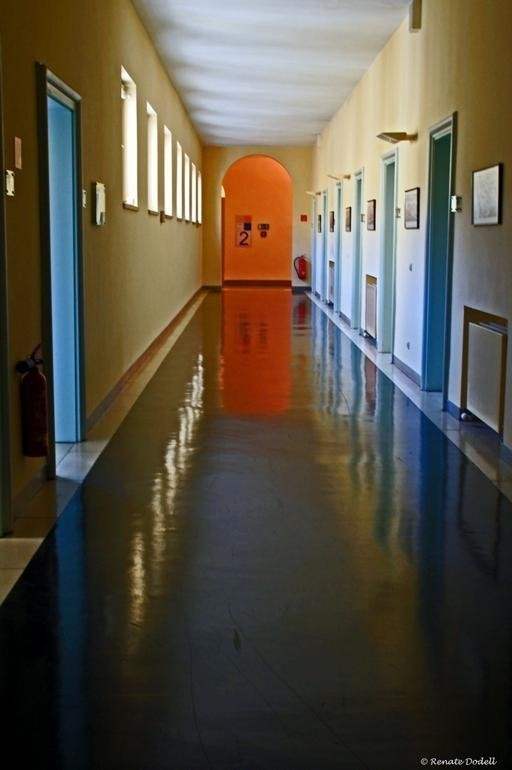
xmin=15 ymin=339 xmax=51 ymax=456
xmin=294 ymin=254 xmax=310 ymax=279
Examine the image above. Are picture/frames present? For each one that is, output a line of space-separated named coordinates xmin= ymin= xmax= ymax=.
xmin=403 ymin=186 xmax=421 ymax=231
xmin=344 ymin=205 xmax=352 ymax=232
xmin=365 ymin=198 xmax=376 ymax=232
xmin=471 ymin=162 xmax=504 ymax=226
xmin=316 ymin=213 xmax=322 ymax=234
xmin=328 ymin=209 xmax=335 ymax=234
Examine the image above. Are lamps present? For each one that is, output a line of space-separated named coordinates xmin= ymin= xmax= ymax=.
xmin=376 ymin=127 xmax=422 ymax=144
